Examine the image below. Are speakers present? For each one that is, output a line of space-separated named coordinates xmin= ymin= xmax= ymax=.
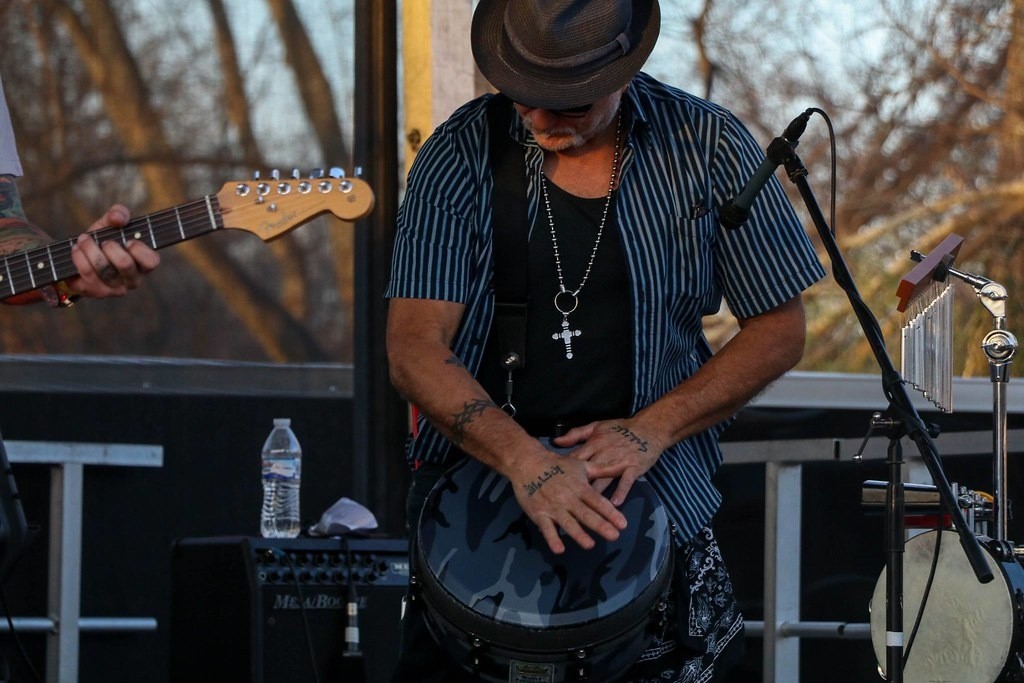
xmin=170 ymin=535 xmax=410 ymax=683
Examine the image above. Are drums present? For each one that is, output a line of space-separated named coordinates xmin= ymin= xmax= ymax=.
xmin=414 ymin=434 xmax=680 ymax=683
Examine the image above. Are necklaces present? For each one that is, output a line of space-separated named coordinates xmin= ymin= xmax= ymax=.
xmin=541 ymin=108 xmax=623 ymax=359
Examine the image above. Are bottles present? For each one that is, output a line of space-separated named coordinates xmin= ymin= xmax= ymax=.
xmin=261 ymin=419 xmax=301 ymax=539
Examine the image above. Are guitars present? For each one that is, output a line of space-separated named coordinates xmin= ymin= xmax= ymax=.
xmin=0 ymin=164 xmax=375 ymax=299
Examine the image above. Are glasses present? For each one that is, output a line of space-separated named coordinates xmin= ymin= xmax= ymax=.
xmin=551 ymin=103 xmax=593 ymax=118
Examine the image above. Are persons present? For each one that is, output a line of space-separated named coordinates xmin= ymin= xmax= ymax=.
xmin=386 ymin=0 xmax=826 ymax=683
xmin=0 ymin=75 xmax=161 ymax=305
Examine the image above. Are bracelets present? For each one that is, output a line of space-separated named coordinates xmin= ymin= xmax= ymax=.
xmin=39 ymin=280 xmax=83 ymax=307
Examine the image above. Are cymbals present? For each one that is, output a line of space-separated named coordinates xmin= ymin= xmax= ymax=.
xmin=869 ymin=522 xmax=1024 ymax=683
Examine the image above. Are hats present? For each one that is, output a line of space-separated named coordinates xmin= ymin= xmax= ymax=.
xmin=470 ymin=0 xmax=660 ymax=110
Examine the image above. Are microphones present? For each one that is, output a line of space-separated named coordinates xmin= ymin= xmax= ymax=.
xmin=342 ymin=581 xmax=364 ymax=677
xmin=719 ymin=108 xmax=814 ymax=230
